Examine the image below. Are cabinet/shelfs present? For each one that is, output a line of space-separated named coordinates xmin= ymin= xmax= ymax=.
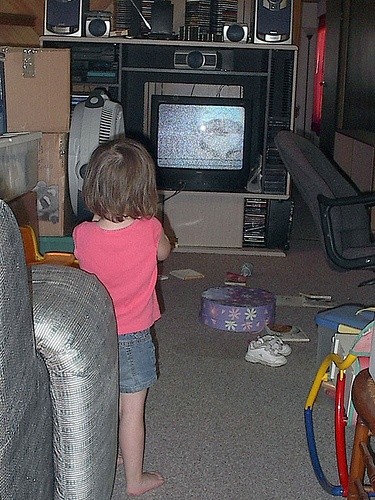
xmin=39 ymin=34 xmax=298 ymax=258
xmin=334 ymin=128 xmax=375 ymax=198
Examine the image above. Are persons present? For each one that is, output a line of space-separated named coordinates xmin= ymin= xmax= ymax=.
xmin=71 ymin=139 xmax=165 ymax=495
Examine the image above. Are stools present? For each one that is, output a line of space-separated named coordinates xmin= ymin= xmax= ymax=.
xmin=348 ymin=367 xmax=375 ymax=500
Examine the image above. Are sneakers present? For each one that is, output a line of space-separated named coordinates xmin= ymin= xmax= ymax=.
xmin=245 ymin=340 xmax=287 ymax=367
xmin=257 ymin=334 xmax=292 ymax=356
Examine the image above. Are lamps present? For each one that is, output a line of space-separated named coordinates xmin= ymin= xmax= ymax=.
xmin=301 ymin=26 xmax=317 ymax=138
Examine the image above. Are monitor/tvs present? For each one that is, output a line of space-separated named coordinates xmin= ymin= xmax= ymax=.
xmin=150 ymin=94 xmax=251 ymax=191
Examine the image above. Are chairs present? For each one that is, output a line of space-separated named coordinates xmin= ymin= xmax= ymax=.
xmin=275 ymin=130 xmax=375 ymax=289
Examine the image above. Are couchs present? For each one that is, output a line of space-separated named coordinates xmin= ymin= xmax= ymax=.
xmin=0 ymin=196 xmax=119 ymax=500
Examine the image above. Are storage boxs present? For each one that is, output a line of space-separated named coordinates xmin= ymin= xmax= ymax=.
xmin=0 ymin=130 xmax=43 ymax=203
xmin=315 ymin=303 xmax=375 ymax=380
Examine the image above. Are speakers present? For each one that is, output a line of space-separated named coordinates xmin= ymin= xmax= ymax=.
xmin=222 ymin=23 xmax=249 ymax=44
xmin=173 ymin=50 xmax=218 ymax=71
xmin=43 ymin=1 xmax=83 ymax=36
xmin=85 ymin=16 xmax=111 ymax=38
xmin=253 ymin=0 xmax=294 ymax=45
